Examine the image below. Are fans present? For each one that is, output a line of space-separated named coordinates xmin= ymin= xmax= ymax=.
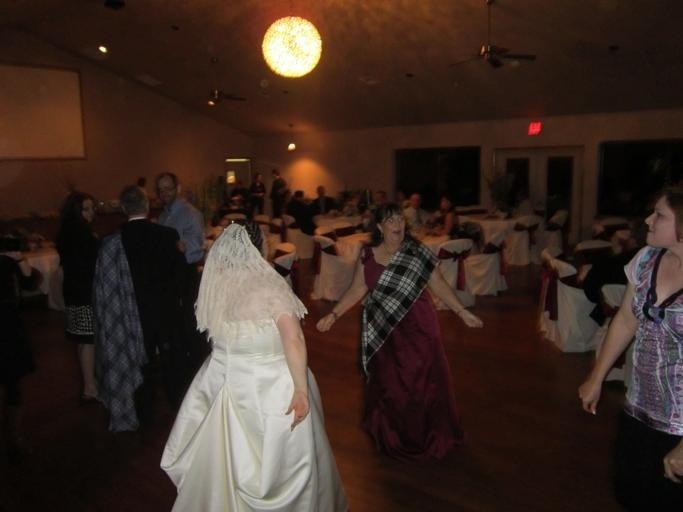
xmin=448 ymin=0 xmax=537 ymax=71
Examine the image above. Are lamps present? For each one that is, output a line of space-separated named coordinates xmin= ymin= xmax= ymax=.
xmin=209 ymin=88 xmax=225 ymax=107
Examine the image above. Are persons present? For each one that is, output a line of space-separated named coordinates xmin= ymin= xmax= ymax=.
xmin=160 ymin=217 xmax=348 ymax=512
xmin=226 ymin=168 xmax=336 ymax=221
xmin=577 ymin=189 xmax=683 ymax=512
xmin=402 ymin=192 xmax=429 ymax=241
xmin=316 ymin=203 xmax=484 ymax=467
xmin=2 ymin=247 xmax=44 ymax=465
xmin=428 ymin=193 xmax=460 ymax=239
xmin=99 ymin=185 xmax=187 ymax=433
xmin=155 ymin=172 xmax=205 ymax=359
xmin=57 ymin=192 xmax=106 ymax=403
xmin=373 ymin=191 xmax=387 ymax=208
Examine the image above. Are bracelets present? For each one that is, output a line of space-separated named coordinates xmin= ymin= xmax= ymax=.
xmin=329 ymin=311 xmax=340 ymax=322
xmin=14 ymin=257 xmax=25 ymax=264
xmin=456 ymin=308 xmax=466 ymax=316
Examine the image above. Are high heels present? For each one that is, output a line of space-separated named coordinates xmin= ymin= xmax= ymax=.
xmin=81 ymin=392 xmax=102 ymax=405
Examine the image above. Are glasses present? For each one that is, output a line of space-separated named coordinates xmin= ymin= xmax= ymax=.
xmin=384 ymin=216 xmax=404 ymax=225
xmin=157 ymin=185 xmax=176 ymax=193
